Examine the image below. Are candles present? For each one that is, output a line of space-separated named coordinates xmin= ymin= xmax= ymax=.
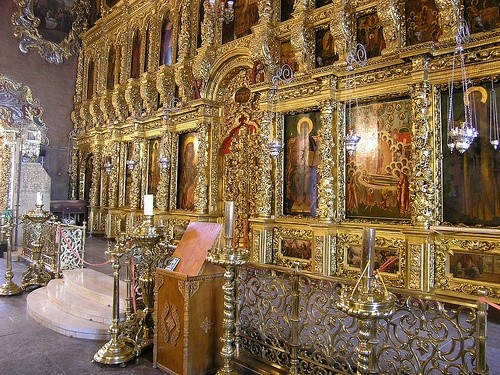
xmin=37 ymin=192 xmax=45 ymax=204
xmin=144 ymin=194 xmax=154 ymax=214
xmin=361 ymin=226 xmax=376 ymax=277
xmin=225 ymin=201 xmax=235 ymax=237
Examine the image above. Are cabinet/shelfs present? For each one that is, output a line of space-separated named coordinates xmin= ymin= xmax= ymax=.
xmin=49 ymin=200 xmax=87 ymax=217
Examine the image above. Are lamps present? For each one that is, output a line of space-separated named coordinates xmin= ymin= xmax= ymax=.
xmin=267 ymin=64 xmax=294 ymax=162
xmin=446 ymin=0 xmax=500 ymax=153
xmin=341 ymin=36 xmax=368 ymax=156
xmin=102 ymin=98 xmax=181 ymax=175
